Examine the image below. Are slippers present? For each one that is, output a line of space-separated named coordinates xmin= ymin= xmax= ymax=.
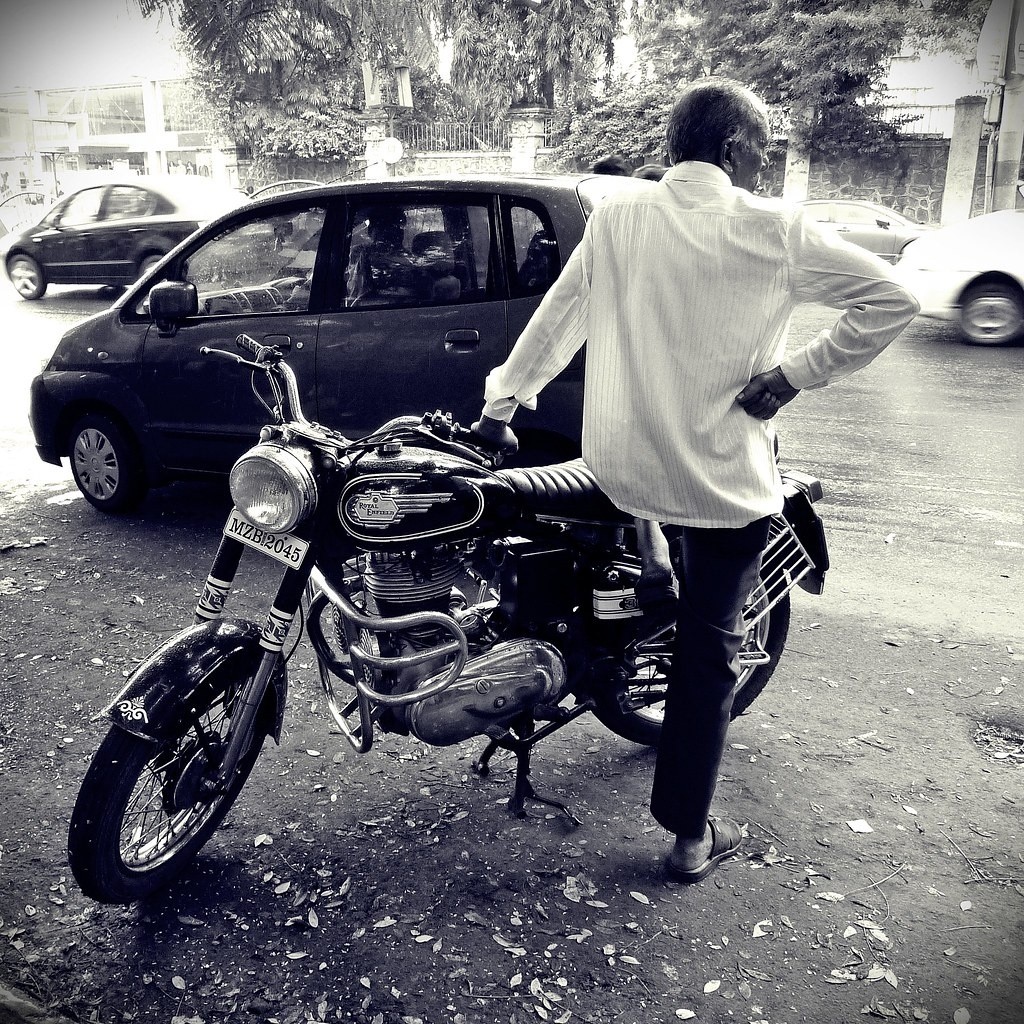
xmin=632 ymin=577 xmax=681 ymax=641
xmin=669 ymin=818 xmax=743 ymax=882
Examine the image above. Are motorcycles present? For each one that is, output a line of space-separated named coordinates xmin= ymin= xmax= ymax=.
xmin=63 ymin=330 xmax=833 ymax=908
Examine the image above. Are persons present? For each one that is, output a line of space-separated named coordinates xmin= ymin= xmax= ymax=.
xmin=471 ymin=74 xmax=921 ymax=885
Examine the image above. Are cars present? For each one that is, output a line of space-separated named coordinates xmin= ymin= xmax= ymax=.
xmin=30 ymin=173 xmax=659 ymax=509
xmin=1 ymin=184 xmax=257 ymax=303
xmin=891 ymin=204 xmax=1023 ymax=339
xmin=798 ymin=194 xmax=932 ymax=269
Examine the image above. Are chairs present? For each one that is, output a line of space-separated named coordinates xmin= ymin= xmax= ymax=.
xmin=411 ymin=231 xmax=460 ymax=302
xmin=518 ymin=230 xmax=552 ymax=293
